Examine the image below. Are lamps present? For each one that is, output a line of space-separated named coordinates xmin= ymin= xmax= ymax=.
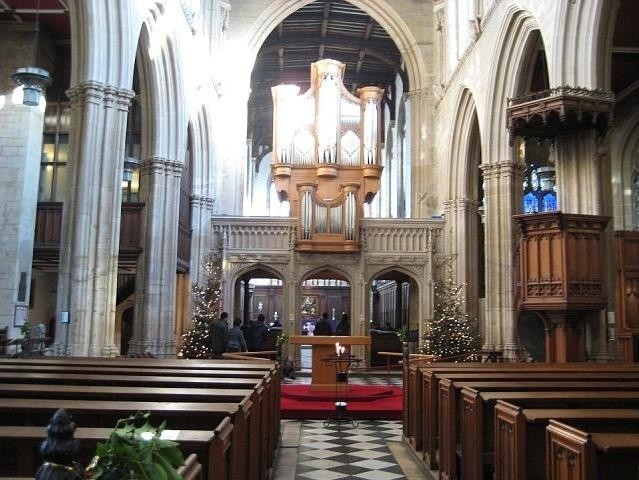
xmin=258 ymin=304 xmax=262 ymax=310
xmin=274 ymin=312 xmax=278 ymax=320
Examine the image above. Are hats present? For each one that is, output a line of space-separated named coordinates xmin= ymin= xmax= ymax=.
xmin=323 ymin=312 xmax=329 ymax=317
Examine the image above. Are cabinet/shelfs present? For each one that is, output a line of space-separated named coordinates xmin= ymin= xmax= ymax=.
xmin=321 ymin=352 xmax=359 ymax=433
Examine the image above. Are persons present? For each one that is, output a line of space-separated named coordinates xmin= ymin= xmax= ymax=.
xmin=336 ymin=314 xmax=349 ymax=335
xmin=246 ymin=314 xmax=267 ymax=351
xmin=380 ymin=321 xmax=394 ymax=331
xmin=272 ymin=320 xmax=280 ymax=327
xmin=313 ymin=312 xmax=332 ymax=335
xmin=209 ymin=312 xmax=228 ymax=354
xmin=226 ymin=318 xmax=248 ymax=352
xmin=241 ymin=320 xmax=250 ymax=340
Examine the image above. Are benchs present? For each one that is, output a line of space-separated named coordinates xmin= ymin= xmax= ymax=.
xmin=1 ymin=353 xmax=282 ymax=480
xmin=402 ymin=342 xmax=639 ymax=479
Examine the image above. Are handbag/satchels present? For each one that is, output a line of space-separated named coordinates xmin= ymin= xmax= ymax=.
xmin=226 ymin=340 xmax=240 ymax=350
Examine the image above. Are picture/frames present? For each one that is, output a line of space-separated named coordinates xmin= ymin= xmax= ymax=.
xmin=13 ymin=305 xmax=30 ymax=328
